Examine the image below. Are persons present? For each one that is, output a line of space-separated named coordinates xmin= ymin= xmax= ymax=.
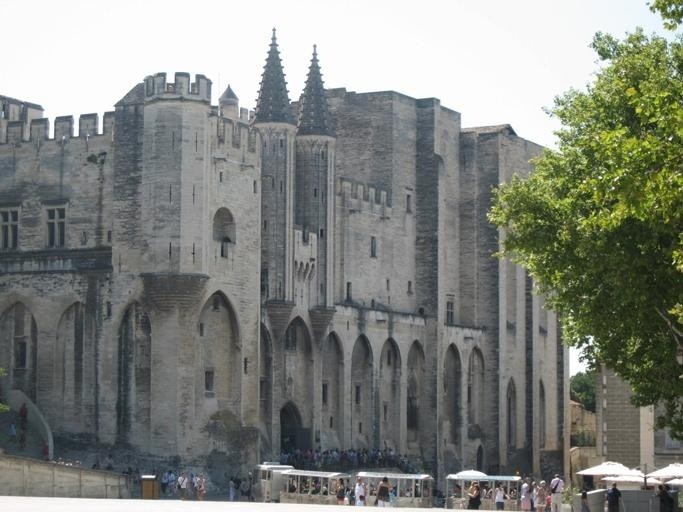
xmin=160 ymin=471 xmax=207 ymax=501
xmin=657 ymin=484 xmax=666 ymax=512
xmin=580 ymin=490 xmax=589 ymax=512
xmin=41 ymin=442 xmax=48 ymax=460
xmin=607 ymin=482 xmax=621 ymax=512
xmin=664 ymin=485 xmax=674 ymax=512
xmin=18 ymin=403 xmax=27 ymax=425
xmin=91 ymin=463 xmax=134 ymax=475
xmin=18 ymin=426 xmax=25 ymax=448
xmin=229 ymin=476 xmax=250 ymax=502
xmin=283 ymin=475 xmax=565 ymax=511
xmin=280 ymin=447 xmax=409 ymax=472
xmin=8 ymin=424 xmax=16 ymax=443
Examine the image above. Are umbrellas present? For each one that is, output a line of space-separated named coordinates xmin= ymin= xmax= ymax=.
xmin=664 ymin=477 xmax=682 ymax=484
xmin=644 ymin=462 xmax=682 ymax=477
xmin=575 ymin=460 xmax=643 ymax=477
xmin=599 ymin=468 xmax=660 ymax=484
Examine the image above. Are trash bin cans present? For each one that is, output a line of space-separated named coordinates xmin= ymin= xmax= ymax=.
xmin=141 ymin=474 xmax=159 ymax=499
